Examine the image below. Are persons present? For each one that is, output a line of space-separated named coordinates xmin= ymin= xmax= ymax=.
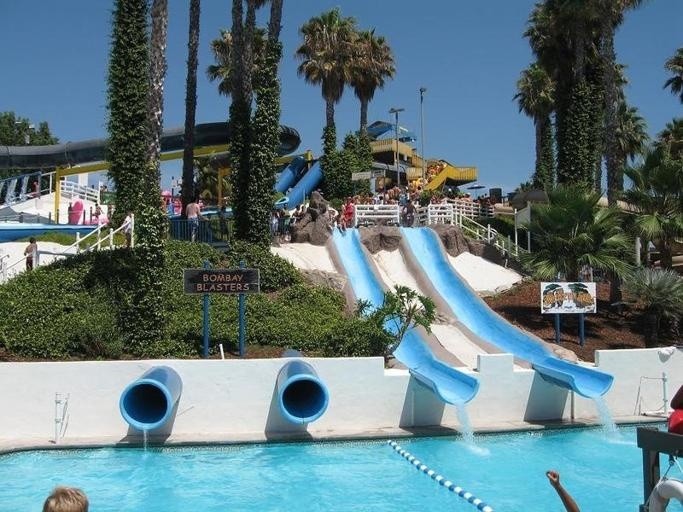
xmin=185 ymin=195 xmax=208 ymax=242
xmin=337 ymin=160 xmax=496 ymax=232
xmin=24 ymin=237 xmax=37 ymax=270
xmin=43 ymin=486 xmax=89 ymax=512
xmin=26 ymin=180 xmax=41 ymax=197
xmin=120 ymin=208 xmax=135 ymax=245
xmin=67 ymin=185 xmax=182 ymax=224
xmin=546 ymin=470 xmax=580 ymax=512
xmin=270 ymin=202 xmax=306 ymax=235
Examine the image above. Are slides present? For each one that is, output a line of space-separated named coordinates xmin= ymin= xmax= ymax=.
xmin=0 ymin=122 xmax=324 ymax=243
xmin=333 ymin=225 xmax=480 ymax=405
xmin=399 ymin=226 xmax=614 ymax=399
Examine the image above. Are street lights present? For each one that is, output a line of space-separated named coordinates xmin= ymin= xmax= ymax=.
xmin=389 ymin=107 xmax=405 ymax=188
xmin=24 ymin=125 xmax=35 ymax=144
xmin=13 ymin=121 xmax=22 ymax=144
xmin=419 ymin=86 xmax=427 ymax=179
